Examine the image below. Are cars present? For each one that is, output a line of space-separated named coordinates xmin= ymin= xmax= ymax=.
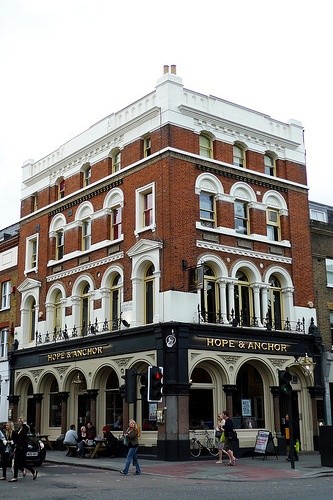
xmin=3 ymin=432 xmax=46 ymax=467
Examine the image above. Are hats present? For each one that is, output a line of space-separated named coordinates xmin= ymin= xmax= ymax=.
xmin=102 ymin=426 xmax=110 ymax=433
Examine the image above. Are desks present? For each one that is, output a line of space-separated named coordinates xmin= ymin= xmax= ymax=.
xmin=37 ymin=434 xmax=53 ymax=450
xmin=90 ymin=440 xmax=123 ymax=459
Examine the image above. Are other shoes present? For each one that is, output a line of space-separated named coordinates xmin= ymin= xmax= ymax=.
xmin=120 ymin=470 xmax=127 ymax=475
xmin=0 ymin=476 xmax=6 ymax=480
xmin=285 ymin=457 xmax=291 ymax=461
xmin=216 ymin=460 xmax=222 ymax=463
xmin=23 ymin=473 xmax=26 ymax=477
xmin=8 ymin=479 xmax=18 ymax=483
xmin=231 ymin=458 xmax=238 ymax=466
xmin=133 ymin=472 xmax=140 ymax=475
xmin=294 ymin=458 xmax=299 ymax=461
xmin=227 ymin=461 xmax=231 ymax=466
xmin=33 ymin=470 xmax=38 ymax=480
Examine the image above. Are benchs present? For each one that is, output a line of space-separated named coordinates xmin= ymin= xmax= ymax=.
xmin=65 ymin=446 xmax=94 ymax=450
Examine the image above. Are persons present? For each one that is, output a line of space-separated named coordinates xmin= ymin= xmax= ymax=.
xmin=77 ymin=426 xmax=89 ymax=458
xmin=215 ymin=411 xmax=236 ymax=466
xmin=8 ymin=417 xmax=38 ymax=482
xmin=120 ymin=420 xmax=141 ymax=475
xmin=0 ymin=421 xmax=26 ymax=480
xmin=86 ymin=421 xmax=96 ymax=440
xmin=103 ymin=426 xmax=119 ymax=458
xmin=63 ymin=425 xmax=78 ymax=445
xmin=285 ymin=414 xmax=299 ymax=462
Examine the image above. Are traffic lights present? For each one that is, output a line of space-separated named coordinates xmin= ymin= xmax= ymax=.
xmin=148 ymin=367 xmax=163 ymax=402
xmin=121 ymin=368 xmax=136 ymax=403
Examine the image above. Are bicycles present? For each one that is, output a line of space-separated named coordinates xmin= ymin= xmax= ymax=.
xmin=189 ymin=426 xmax=219 ymax=458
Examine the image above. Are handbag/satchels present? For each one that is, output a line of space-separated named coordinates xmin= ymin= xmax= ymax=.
xmin=228 ymin=431 xmax=239 ymax=452
xmin=296 ymin=440 xmax=300 ymax=452
xmin=215 ymin=428 xmax=222 ymax=439
xmin=220 ymin=431 xmax=227 ymax=443
xmin=123 ymin=437 xmax=129 ymax=445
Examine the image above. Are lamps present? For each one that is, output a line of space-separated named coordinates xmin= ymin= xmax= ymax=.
xmin=63 ymin=332 xmax=68 ymax=339
xmin=300 ymin=353 xmax=316 ymax=376
xmin=91 ymin=326 xmax=98 ymax=334
xmin=121 ymin=319 xmax=130 ymax=328
xmin=72 ymin=373 xmax=85 ymax=384
